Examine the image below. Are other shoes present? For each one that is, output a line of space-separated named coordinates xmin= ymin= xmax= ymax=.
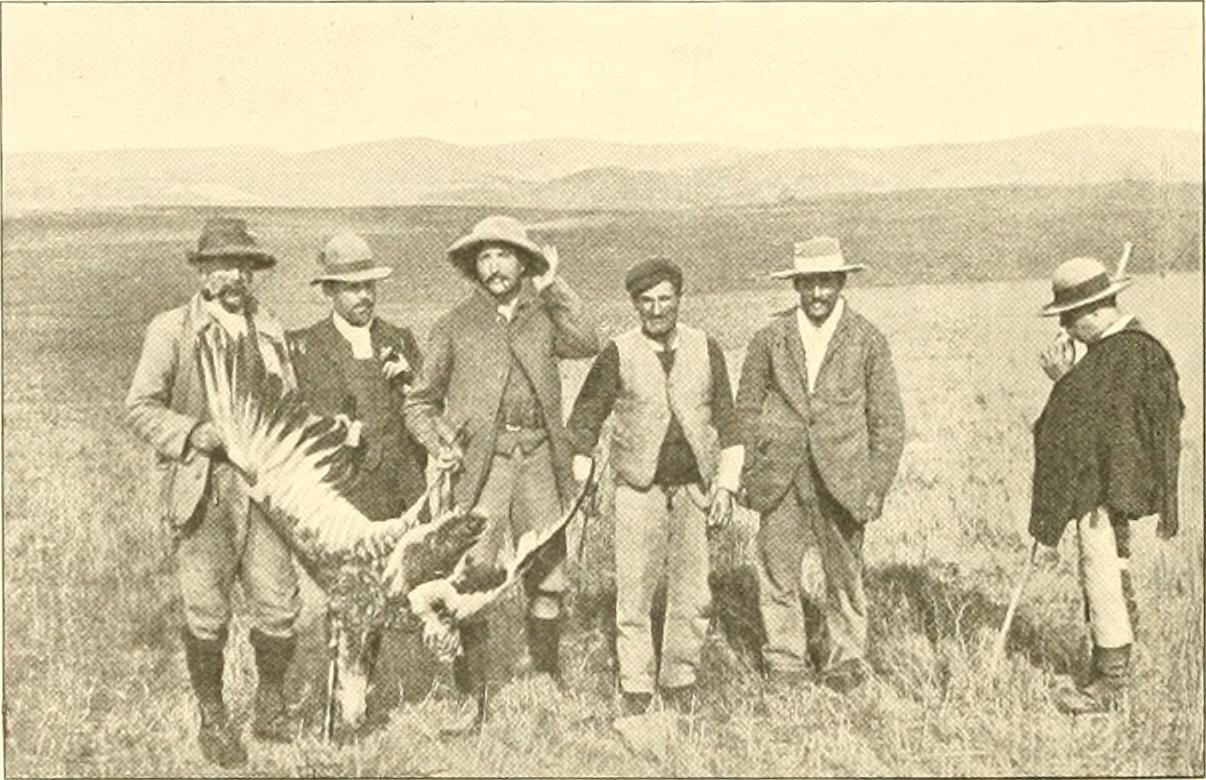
xmin=619 ymin=689 xmax=654 ymax=718
xmin=825 ymin=659 xmax=864 ymax=696
xmin=659 ymin=684 xmax=695 ymax=716
xmin=767 ymin=671 xmax=813 ymax=704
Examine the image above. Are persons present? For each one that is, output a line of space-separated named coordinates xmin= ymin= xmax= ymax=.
xmin=1027 ymin=256 xmax=1185 ymax=715
xmin=122 ymin=217 xmax=906 ymax=771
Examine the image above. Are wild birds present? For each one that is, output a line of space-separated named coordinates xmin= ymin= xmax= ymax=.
xmin=196 ymin=324 xmax=598 ymax=732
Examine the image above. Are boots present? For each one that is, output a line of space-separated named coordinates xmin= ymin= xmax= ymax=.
xmin=250 ymin=629 xmax=295 ymax=742
xmin=1078 ymin=643 xmax=1132 ymax=713
xmin=526 ymin=609 xmax=559 ymax=682
xmin=450 ymin=620 xmax=490 ymax=724
xmin=180 ymin=625 xmax=246 ymax=769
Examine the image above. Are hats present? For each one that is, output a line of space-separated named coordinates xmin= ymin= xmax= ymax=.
xmin=187 ymin=216 xmax=276 ymax=271
xmin=771 ymin=237 xmax=865 ymax=279
xmin=445 ymin=215 xmax=548 ymax=281
xmin=1039 ymin=258 xmax=1133 ymax=317
xmin=310 ymin=232 xmax=393 ymax=284
xmin=624 ymin=255 xmax=684 ymax=296
